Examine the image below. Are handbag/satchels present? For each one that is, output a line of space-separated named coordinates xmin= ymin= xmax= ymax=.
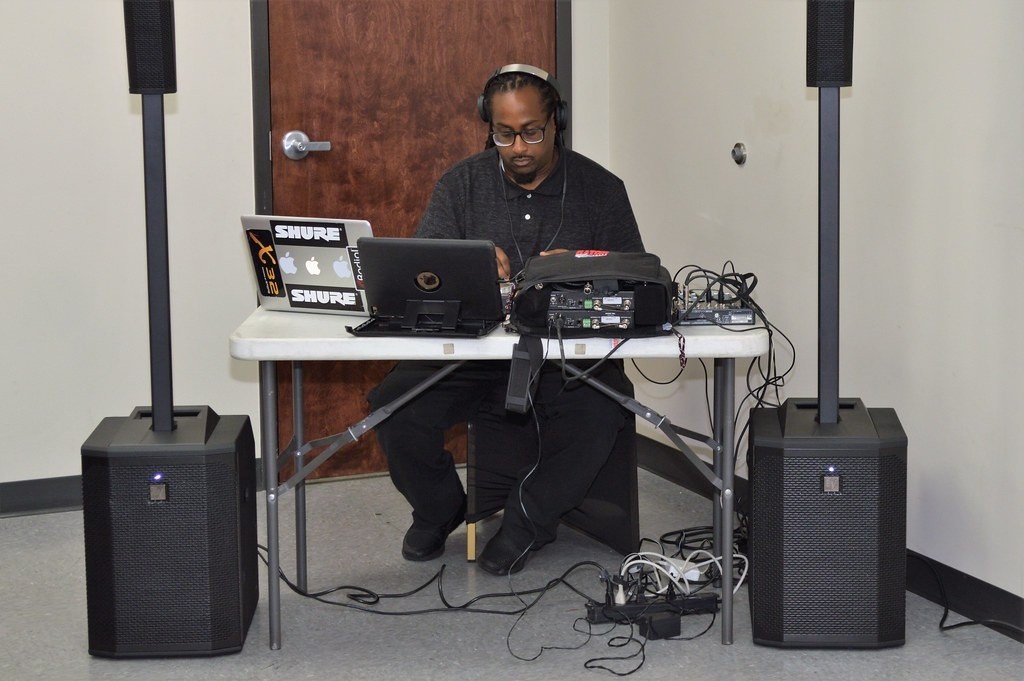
xmin=505 ymin=250 xmax=672 ymax=413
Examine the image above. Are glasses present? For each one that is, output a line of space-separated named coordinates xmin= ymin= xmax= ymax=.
xmin=489 ymin=107 xmax=555 ymax=147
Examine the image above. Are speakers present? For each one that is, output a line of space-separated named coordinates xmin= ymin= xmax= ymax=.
xmin=80 ymin=406 xmax=260 ymax=659
xmin=748 ymin=397 xmax=908 ymax=649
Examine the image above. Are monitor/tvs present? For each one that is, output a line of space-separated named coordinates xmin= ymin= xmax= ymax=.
xmin=356 ymin=236 xmax=504 ymax=322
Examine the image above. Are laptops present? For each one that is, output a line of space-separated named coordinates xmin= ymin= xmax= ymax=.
xmin=240 ymin=215 xmax=373 ymax=317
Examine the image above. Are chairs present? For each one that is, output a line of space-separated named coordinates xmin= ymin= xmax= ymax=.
xmin=467 ymin=359 xmax=640 ymax=561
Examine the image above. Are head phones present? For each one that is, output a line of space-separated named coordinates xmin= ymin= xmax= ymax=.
xmin=477 ymin=64 xmax=568 ymax=133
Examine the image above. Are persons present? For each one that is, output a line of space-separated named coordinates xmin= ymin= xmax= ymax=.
xmin=368 ymin=63 xmax=646 ymax=577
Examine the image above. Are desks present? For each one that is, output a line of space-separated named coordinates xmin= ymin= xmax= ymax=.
xmin=224 ymin=291 xmax=771 ymax=646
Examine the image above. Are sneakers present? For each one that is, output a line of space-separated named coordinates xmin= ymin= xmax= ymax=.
xmin=401 ymin=512 xmax=465 ymax=562
xmin=477 ymin=524 xmax=530 ymax=576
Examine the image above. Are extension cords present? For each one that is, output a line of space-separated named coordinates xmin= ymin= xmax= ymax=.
xmin=585 ymin=592 xmax=721 ymax=623
